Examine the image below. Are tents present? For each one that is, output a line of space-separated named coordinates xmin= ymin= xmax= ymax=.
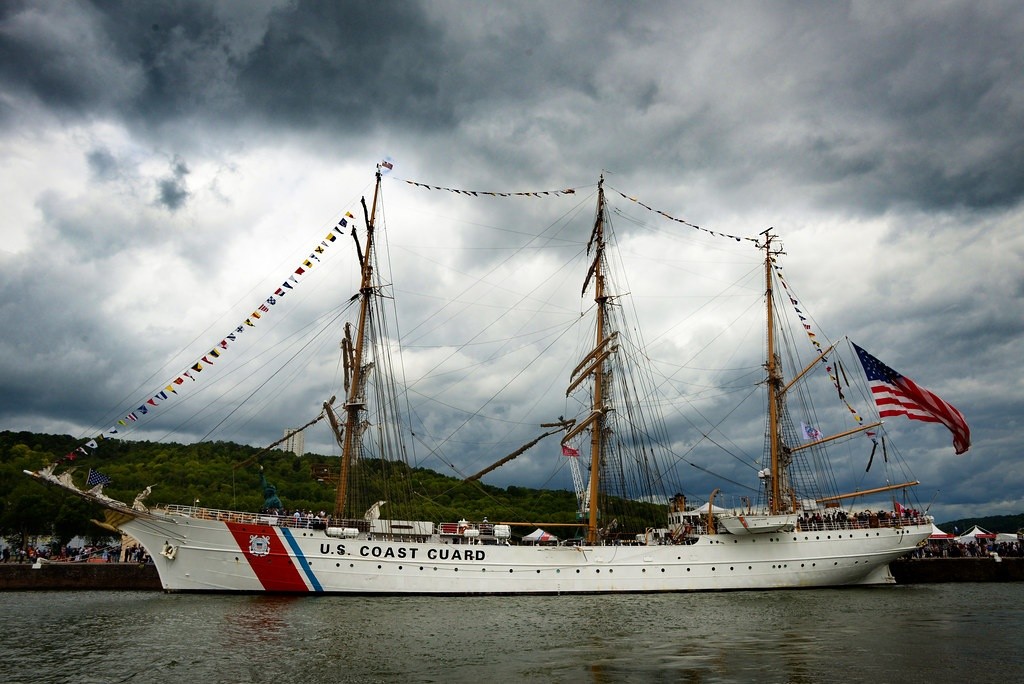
xmin=928 ymin=523 xmax=954 ymax=540
xmin=522 ymin=528 xmax=558 ymax=546
xmin=959 ymin=526 xmax=1003 ymax=557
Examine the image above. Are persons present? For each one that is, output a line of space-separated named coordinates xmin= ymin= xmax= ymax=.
xmin=1 ymin=542 xmax=53 ymax=564
xmin=798 ymin=508 xmax=920 ymax=531
xmin=124 ymin=544 xmax=145 ymax=563
xmin=293 ymin=508 xmax=326 ymax=529
xmin=105 ymin=545 xmax=122 ymax=563
xmin=913 ymin=540 xmax=1024 ymax=558
xmin=61 ymin=545 xmax=67 ymax=562
xmin=71 ymin=543 xmax=99 ymax=561
xmin=261 ymin=508 xmax=287 ymax=528
xmin=683 ymin=514 xmax=718 ymax=535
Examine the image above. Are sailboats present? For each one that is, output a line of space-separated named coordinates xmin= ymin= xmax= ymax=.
xmin=24 ymin=160 xmax=972 ymax=599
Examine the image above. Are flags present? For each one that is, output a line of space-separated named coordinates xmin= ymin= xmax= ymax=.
xmin=771 ymin=255 xmax=877 ymax=441
xmin=848 ymin=339 xmax=973 ymax=456
xmin=562 ymin=445 xmax=580 ymax=456
xmin=895 ymin=502 xmax=914 ymax=517
xmin=88 ymin=470 xmax=111 ymax=489
xmin=801 ymin=420 xmax=824 ymax=442
xmin=54 ymin=206 xmax=357 ymax=460
